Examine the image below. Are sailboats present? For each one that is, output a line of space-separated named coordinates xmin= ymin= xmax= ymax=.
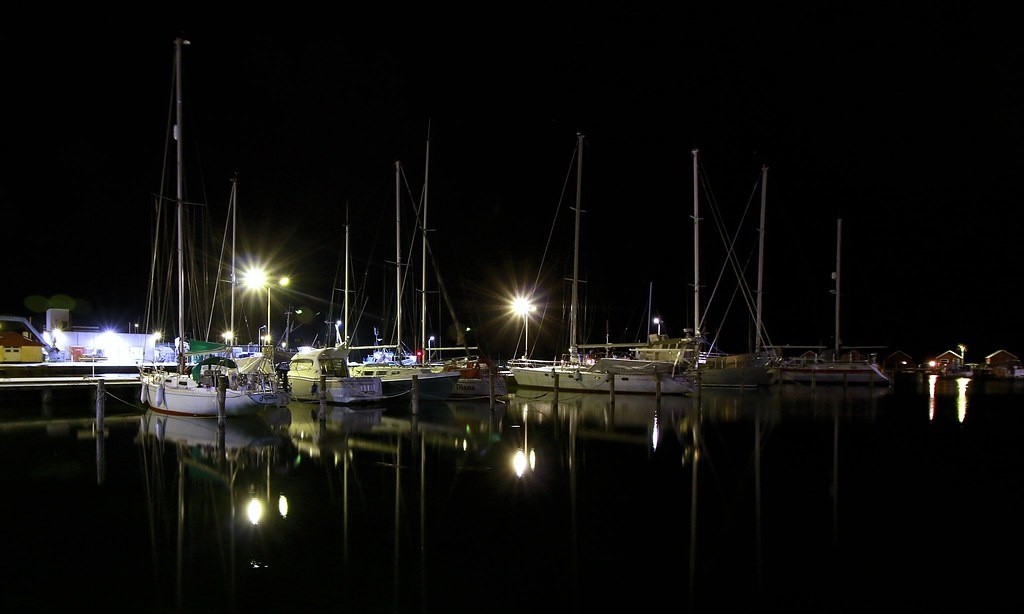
xmin=1 ymin=37 xmax=787 ymax=419
xmin=758 ymin=219 xmax=896 ymax=389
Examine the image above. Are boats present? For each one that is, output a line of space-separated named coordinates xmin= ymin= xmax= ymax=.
xmin=941 ymin=362 xmax=982 ymax=379
